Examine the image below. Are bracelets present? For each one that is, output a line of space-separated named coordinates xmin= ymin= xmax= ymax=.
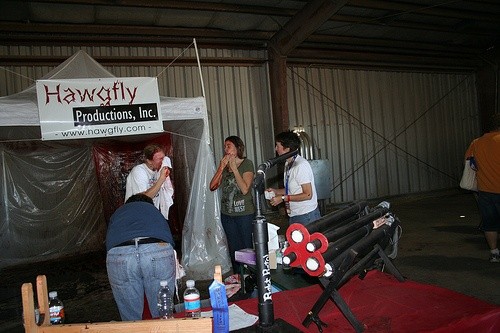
xmin=288 ymin=195 xmax=290 ymax=201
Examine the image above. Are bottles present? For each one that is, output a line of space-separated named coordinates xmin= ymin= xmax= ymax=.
xmin=23 ymin=292 xmax=39 ymax=328
xmin=49 ymin=292 xmax=64 ymax=325
xmin=184 ymin=280 xmax=201 ymax=319
xmin=157 ymin=281 xmax=174 ymax=319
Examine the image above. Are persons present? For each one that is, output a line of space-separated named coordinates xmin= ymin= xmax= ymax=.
xmin=464 ymin=113 xmax=500 ymax=264
xmin=125 ymin=145 xmax=174 ymax=220
xmin=107 ymin=194 xmax=176 ymax=321
xmin=264 ymin=132 xmax=320 ymax=274
xmin=209 ymin=136 xmax=255 ymax=273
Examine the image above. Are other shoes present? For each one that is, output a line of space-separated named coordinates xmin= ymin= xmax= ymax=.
xmin=489 ymin=252 xmax=500 ymax=263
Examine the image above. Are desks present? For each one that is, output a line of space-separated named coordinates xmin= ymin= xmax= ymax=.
xmin=239 ymin=262 xmax=319 ymax=294
xmin=141 ymin=266 xmax=500 ymax=333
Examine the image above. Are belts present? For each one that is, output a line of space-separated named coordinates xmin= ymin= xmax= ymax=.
xmin=114 ymin=237 xmax=169 ymax=247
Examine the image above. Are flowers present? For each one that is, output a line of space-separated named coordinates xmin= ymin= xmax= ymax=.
xmin=293 ymin=127 xmax=306 ymax=137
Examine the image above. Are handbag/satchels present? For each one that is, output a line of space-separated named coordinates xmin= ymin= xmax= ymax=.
xmin=460 ymin=154 xmax=478 ymax=191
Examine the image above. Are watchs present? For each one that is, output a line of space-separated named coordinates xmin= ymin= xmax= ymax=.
xmin=281 ymin=194 xmax=285 ymax=202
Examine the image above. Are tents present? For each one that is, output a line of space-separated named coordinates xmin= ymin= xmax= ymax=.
xmin=0 ymin=49 xmax=234 ymax=285
